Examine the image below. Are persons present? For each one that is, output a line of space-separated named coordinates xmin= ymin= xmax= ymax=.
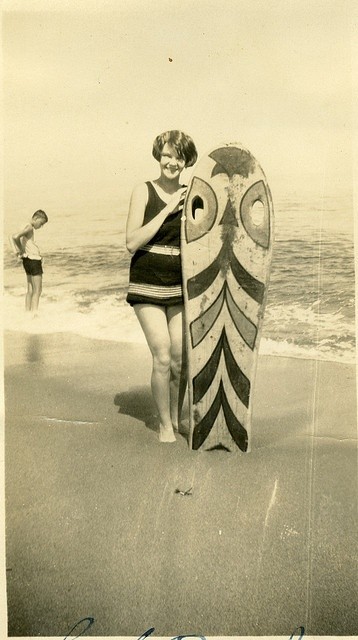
xmin=126 ymin=130 xmax=204 ymax=444
xmin=9 ymin=210 xmax=48 ymax=319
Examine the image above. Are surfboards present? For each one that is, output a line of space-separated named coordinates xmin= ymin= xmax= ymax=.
xmin=181 ymin=143 xmax=276 ymax=453
